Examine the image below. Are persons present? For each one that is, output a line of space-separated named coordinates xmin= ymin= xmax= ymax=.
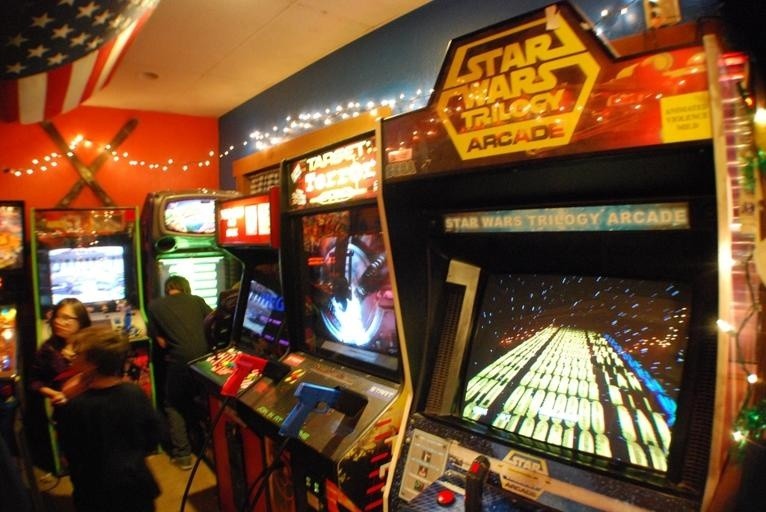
xmin=145 ymin=276 xmax=213 ymax=470
xmin=48 ymin=321 xmax=164 ymax=512
xmin=27 ymin=296 xmax=95 ymax=470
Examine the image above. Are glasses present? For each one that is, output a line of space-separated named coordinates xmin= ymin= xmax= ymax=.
xmin=52 ymin=314 xmax=76 ymax=323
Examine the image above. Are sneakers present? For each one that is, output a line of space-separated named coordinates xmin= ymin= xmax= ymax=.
xmin=172 ymin=453 xmax=194 ymax=469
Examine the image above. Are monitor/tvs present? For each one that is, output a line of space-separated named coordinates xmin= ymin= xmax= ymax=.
xmin=241 ymin=256 xmax=289 ymax=347
xmin=296 ymin=205 xmax=400 ymax=373
xmin=156 ymin=256 xmax=227 ymax=309
xmin=45 ymin=244 xmax=129 ymax=307
xmin=459 ymin=264 xmax=699 ymax=480
